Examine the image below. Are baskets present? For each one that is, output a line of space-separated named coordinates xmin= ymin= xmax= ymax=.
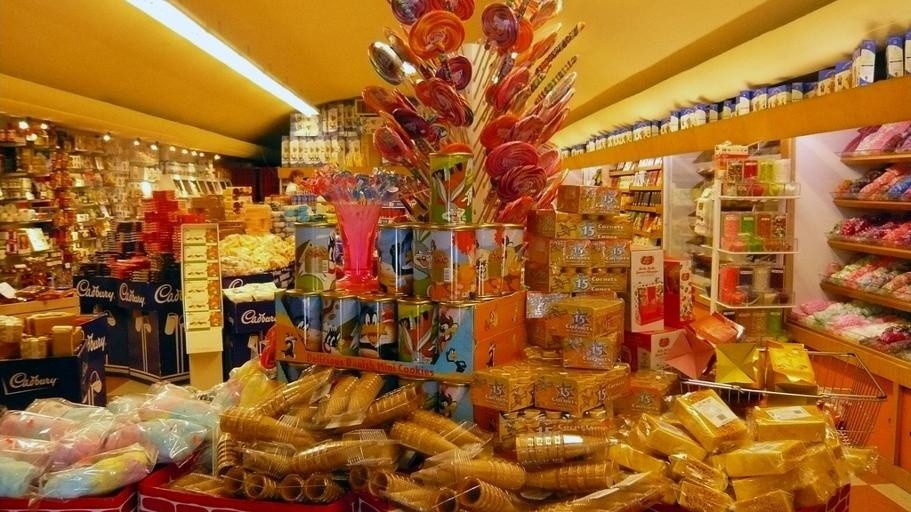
xmin=680 ymin=351 xmax=887 ymax=448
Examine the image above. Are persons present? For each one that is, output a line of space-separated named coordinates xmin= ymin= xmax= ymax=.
xmin=284 ymin=170 xmax=308 ymax=196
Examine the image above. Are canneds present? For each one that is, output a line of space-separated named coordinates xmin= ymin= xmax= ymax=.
xmin=275 ymin=152 xmax=525 ymax=425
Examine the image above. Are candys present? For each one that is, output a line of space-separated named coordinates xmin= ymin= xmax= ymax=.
xmin=793 ymin=120 xmax=911 ymax=361
xmin=294 ymin=0 xmax=587 ymax=222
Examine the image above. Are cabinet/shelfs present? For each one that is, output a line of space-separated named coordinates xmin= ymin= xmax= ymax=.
xmin=561 ymin=76 xmax=911 ymax=473
